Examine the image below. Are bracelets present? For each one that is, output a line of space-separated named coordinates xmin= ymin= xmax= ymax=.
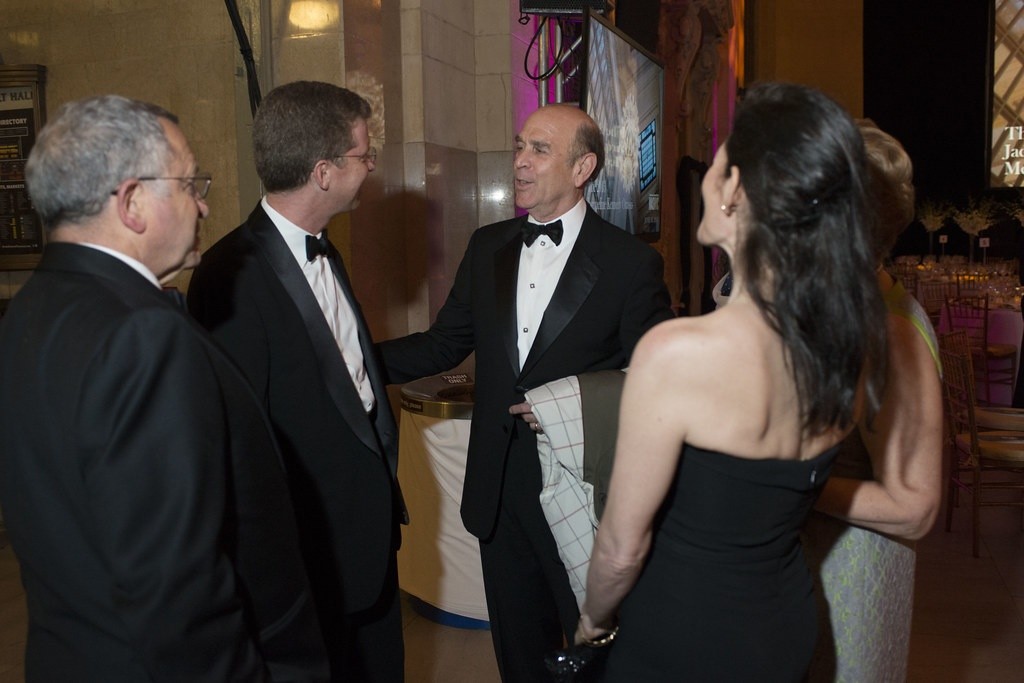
xmin=578 ymin=612 xmax=620 ymax=648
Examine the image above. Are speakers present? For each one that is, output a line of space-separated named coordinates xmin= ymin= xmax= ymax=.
xmin=519 ymin=0 xmax=610 ymax=16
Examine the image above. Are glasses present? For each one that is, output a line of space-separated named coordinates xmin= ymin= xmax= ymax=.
xmin=111 ymin=172 xmax=212 ymax=200
xmin=311 ymin=147 xmax=376 ymax=172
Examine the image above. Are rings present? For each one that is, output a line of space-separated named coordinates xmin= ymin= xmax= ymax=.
xmin=534 ymin=421 xmax=539 ymax=431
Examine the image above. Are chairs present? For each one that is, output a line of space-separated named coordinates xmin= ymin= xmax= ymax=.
xmin=937 ymin=323 xmax=1024 ymax=507
xmin=888 ymin=261 xmax=1024 ymax=329
xmin=943 ymin=291 xmax=1018 ymax=407
xmin=938 ymin=347 xmax=1024 ymax=557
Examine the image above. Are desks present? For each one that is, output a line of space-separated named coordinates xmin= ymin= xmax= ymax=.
xmin=940 ymin=301 xmax=1024 ymax=403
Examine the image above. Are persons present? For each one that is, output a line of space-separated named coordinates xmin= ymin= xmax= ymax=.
xmin=0 ymin=95 xmax=329 ymax=682
xmin=572 ymin=83 xmax=890 ymax=683
xmin=186 ymin=81 xmax=410 ymax=683
xmin=375 ymin=103 xmax=674 ymax=683
xmin=811 ymin=121 xmax=945 ymax=683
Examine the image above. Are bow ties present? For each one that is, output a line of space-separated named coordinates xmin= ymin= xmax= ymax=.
xmin=521 ymin=219 xmax=563 ymax=248
xmin=306 ymin=228 xmax=328 ymax=262
xmin=163 ymin=286 xmax=183 ymax=308
